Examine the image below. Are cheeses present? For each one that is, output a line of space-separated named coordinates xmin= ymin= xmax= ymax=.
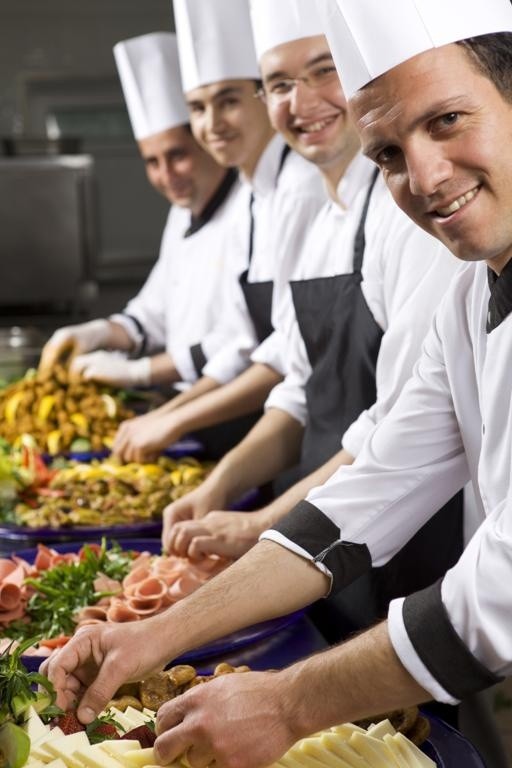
xmin=21 ymin=704 xmax=441 ymax=768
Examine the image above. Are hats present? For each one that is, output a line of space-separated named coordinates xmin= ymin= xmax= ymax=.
xmin=250 ymin=1 xmax=324 ymax=63
xmin=321 ymin=0 xmax=512 ymax=104
xmin=172 ymin=0 xmax=261 ymax=94
xmin=113 ymin=31 xmax=189 ymax=141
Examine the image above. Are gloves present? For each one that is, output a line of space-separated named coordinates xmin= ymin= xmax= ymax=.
xmin=36 ymin=320 xmax=150 ymax=390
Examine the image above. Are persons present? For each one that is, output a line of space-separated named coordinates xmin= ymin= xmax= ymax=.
xmin=116 ymin=78 xmax=331 ymax=512
xmin=37 ymin=33 xmax=510 ymax=766
xmin=39 ymin=121 xmax=264 ymax=456
xmin=162 ymin=32 xmax=479 ymax=650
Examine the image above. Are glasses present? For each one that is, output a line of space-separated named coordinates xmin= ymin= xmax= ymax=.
xmin=253 ymin=68 xmax=338 ymax=104
